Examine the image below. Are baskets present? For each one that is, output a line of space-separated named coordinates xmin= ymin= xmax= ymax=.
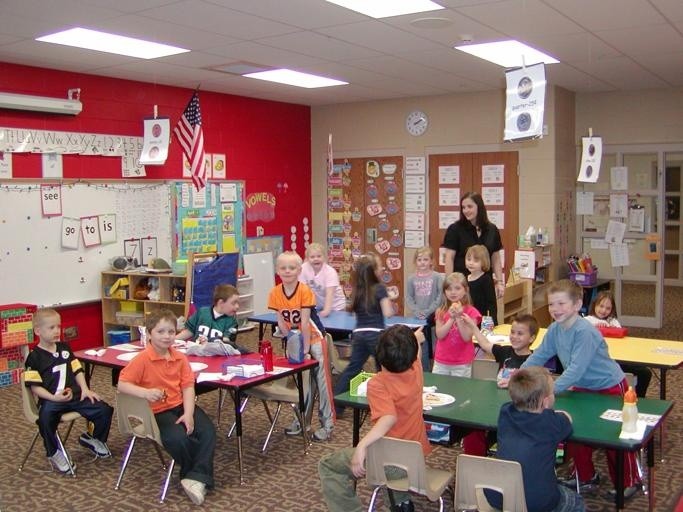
xmin=350 ymin=372 xmax=377 ymax=397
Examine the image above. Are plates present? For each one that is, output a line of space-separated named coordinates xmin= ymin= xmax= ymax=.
xmin=145 ymin=269 xmax=171 ymax=274
xmin=422 ymin=390 xmax=455 ymax=408
xmin=116 ymin=350 xmax=140 ymax=361
xmin=189 ymin=362 xmax=208 ymax=372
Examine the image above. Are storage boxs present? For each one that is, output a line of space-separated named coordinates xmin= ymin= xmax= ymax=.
xmin=115 ymin=311 xmax=145 ymax=328
xmin=104 ymin=278 xmax=129 ymax=300
xmin=566 ymin=268 xmax=598 ymax=287
xmin=117 ymin=300 xmax=140 ymax=313
xmin=106 ymin=327 xmax=131 ymax=345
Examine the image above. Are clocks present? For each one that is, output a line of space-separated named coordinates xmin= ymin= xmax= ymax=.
xmin=404 ymin=108 xmax=431 ymax=138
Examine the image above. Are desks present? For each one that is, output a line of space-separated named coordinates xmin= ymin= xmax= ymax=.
xmin=468 ymin=322 xmax=683 ymax=462
xmin=72 ymin=337 xmax=321 ymax=485
xmin=569 ymin=278 xmax=611 ymax=318
xmin=331 ymin=371 xmax=678 ymax=511
xmin=244 ymin=310 xmax=434 ymax=378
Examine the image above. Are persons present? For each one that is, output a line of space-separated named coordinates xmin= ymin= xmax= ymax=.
xmin=117 ymin=308 xmax=216 ymax=507
xmin=583 ymin=288 xmax=652 ymax=398
xmin=406 ymin=246 xmax=443 ymax=360
xmin=23 ymin=307 xmax=113 ymax=475
xmin=175 ymin=284 xmax=240 ymax=345
xmin=432 ymin=272 xmax=483 ymax=378
xmin=298 ymin=243 xmax=348 ymax=341
xmin=497 ymin=278 xmax=643 ymax=502
xmin=463 ymin=244 xmax=498 ymax=329
xmin=333 ymin=252 xmax=394 ymax=420
xmin=484 ymin=365 xmax=587 ymax=512
xmin=318 ymin=324 xmax=432 ymax=512
xmin=441 ymin=191 xmax=505 ymax=298
xmin=460 ymin=312 xmax=539 ymax=445
xmin=268 ymin=251 xmax=336 ymax=443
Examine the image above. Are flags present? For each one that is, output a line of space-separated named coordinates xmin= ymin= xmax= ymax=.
xmin=172 ymin=90 xmax=208 ymax=193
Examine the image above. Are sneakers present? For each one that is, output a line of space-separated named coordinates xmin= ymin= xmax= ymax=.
xmin=567 ymin=472 xmax=599 ymax=487
xmin=314 ymin=427 xmax=330 ymax=440
xmin=47 ymin=448 xmax=77 ymax=474
xmin=181 ymin=478 xmax=208 ymax=505
xmin=285 ymin=423 xmax=311 ymax=435
xmin=79 ymin=431 xmax=110 ymax=459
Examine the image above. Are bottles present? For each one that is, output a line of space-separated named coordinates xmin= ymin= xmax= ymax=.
xmin=620 ymin=383 xmax=639 ymax=435
xmin=543 ymin=226 xmax=549 ymax=244
xmin=537 ymin=228 xmax=543 ymax=242
xmin=260 ymin=340 xmax=273 ymax=373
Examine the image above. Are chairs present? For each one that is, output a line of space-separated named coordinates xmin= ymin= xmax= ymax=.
xmin=16 ymin=370 xmax=103 ymax=479
xmin=324 ymin=330 xmax=357 ymax=378
xmin=364 ymin=434 xmax=454 ymax=512
xmin=223 ymin=351 xmax=314 ymax=454
xmin=111 ymin=386 xmax=182 ymax=504
xmin=453 ymin=453 xmax=531 ymax=512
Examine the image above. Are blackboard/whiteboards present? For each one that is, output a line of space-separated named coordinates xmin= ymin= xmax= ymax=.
xmin=0 ymin=178 xmax=246 ymax=312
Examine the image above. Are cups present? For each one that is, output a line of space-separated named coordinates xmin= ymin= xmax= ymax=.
xmin=481 ymin=316 xmax=495 ymax=336
xmin=173 ymin=286 xmax=184 ymax=303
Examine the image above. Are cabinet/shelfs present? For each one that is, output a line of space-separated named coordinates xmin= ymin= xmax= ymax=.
xmin=100 ymin=246 xmax=240 ymax=347
xmin=494 ymin=241 xmax=554 ymax=326
